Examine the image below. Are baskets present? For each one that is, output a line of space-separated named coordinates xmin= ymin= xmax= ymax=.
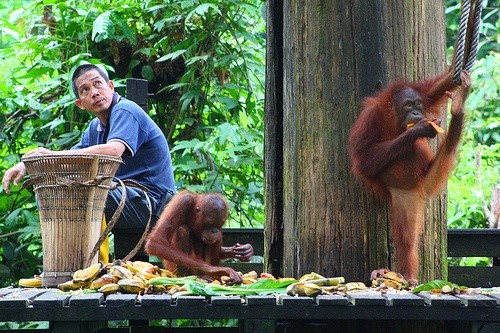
xmin=20 ymin=155 xmax=124 ymax=287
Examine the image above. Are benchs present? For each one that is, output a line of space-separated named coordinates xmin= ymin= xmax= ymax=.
xmin=1 ymin=286 xmax=500 ymax=333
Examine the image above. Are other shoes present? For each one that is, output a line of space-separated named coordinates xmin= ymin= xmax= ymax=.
xmin=19 ymin=271 xmax=44 ymax=288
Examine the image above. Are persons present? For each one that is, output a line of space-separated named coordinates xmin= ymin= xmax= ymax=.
xmin=2 ymin=64 xmax=176 ymax=262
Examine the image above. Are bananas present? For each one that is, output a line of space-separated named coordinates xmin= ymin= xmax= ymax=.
xmin=19 ymin=260 xmax=184 ymax=293
xmin=373 ymin=272 xmax=408 ymax=290
xmin=213 ymin=270 xmax=341 ymax=297
